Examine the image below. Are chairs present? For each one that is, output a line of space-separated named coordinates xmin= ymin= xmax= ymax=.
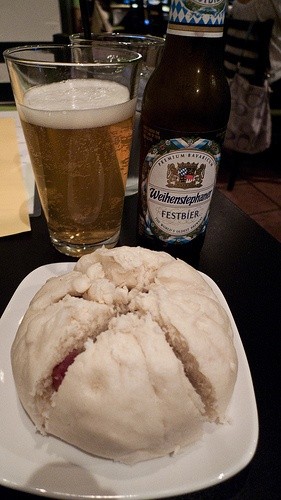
xmin=221 ymin=13 xmax=281 ymax=192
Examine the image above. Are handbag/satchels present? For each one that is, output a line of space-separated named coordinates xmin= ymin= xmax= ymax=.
xmin=225 ymin=71 xmax=275 ymax=155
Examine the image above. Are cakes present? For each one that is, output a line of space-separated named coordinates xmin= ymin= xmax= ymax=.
xmin=9 ymin=247 xmax=237 ymax=461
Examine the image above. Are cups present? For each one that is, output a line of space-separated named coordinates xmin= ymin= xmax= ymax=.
xmin=3 ymin=32 xmax=165 ymax=257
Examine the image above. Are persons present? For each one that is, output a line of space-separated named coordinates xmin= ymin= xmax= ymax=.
xmin=224 ymin=0 xmax=281 ymax=109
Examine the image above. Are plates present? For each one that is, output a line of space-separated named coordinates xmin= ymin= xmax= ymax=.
xmin=0 ymin=262 xmax=259 ymax=500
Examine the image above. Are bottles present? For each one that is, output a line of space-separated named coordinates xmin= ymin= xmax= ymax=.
xmin=138 ymin=0 xmax=231 ymax=265
